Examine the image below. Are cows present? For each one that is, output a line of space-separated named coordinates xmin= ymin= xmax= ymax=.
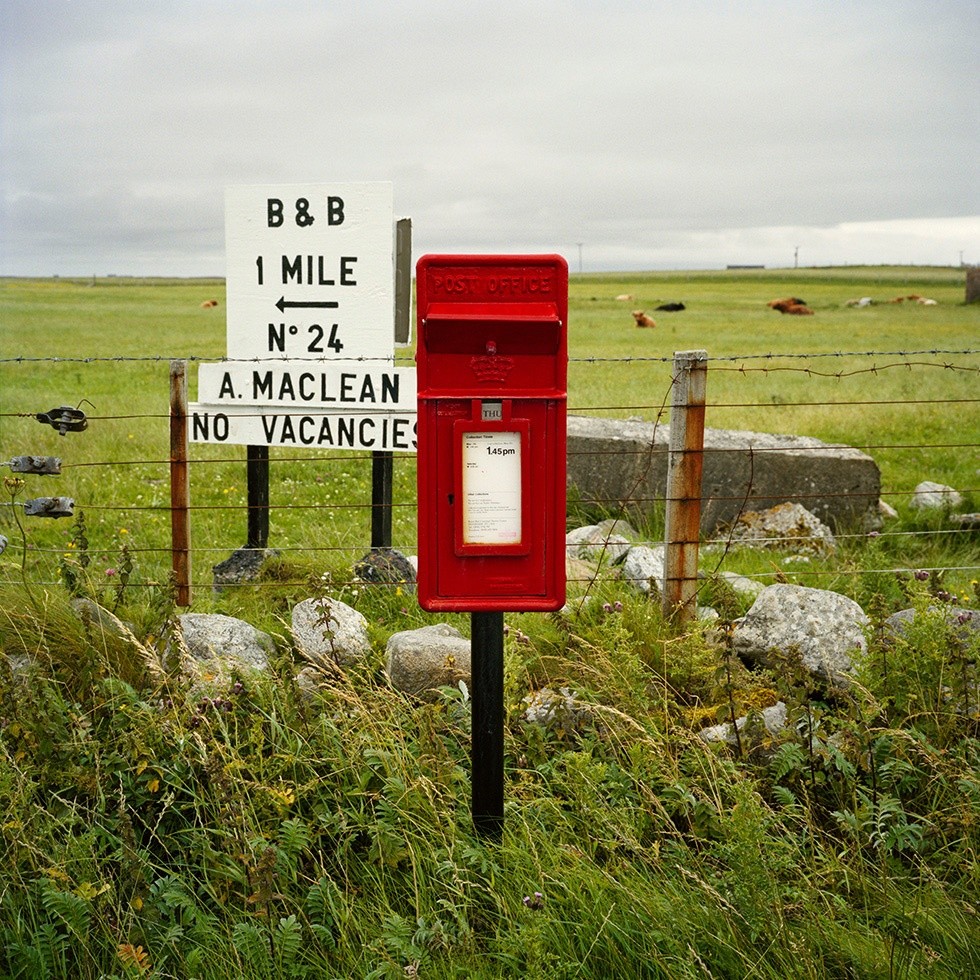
xmin=632 ymin=309 xmax=655 ymax=326
xmin=767 ymin=297 xmax=815 ymax=315
